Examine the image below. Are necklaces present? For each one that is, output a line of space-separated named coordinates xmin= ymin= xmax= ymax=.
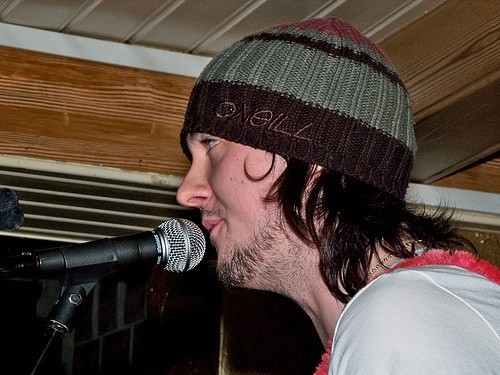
xmin=338 ymin=240 xmax=423 ymax=312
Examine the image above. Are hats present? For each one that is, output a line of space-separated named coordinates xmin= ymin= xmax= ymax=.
xmin=177 ymin=16 xmax=418 ymax=199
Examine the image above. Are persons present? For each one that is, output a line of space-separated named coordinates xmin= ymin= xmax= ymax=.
xmin=176 ymin=15 xmax=500 ymax=375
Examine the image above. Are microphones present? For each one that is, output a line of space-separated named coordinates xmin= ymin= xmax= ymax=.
xmin=0 ymin=218 xmax=206 ymax=274
